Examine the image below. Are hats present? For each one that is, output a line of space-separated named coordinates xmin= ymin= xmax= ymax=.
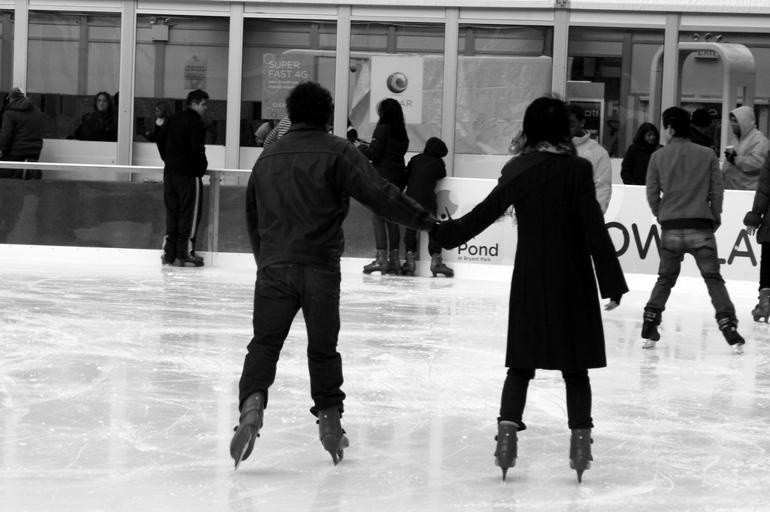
xmin=692 ymin=109 xmax=712 ymax=128
xmin=8 ymin=88 xmax=24 ymax=100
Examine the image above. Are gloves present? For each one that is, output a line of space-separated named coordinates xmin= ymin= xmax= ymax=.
xmin=725 ymin=151 xmax=736 ymax=164
xmin=610 ymin=293 xmax=622 ymax=305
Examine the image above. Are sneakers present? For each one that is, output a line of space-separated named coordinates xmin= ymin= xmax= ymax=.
xmin=316 ymin=408 xmax=349 ymax=451
xmin=231 ymin=392 xmax=264 ymax=460
xmin=431 ymin=253 xmax=453 ymax=273
xmin=364 ymin=250 xmax=390 ymax=274
xmin=401 ymin=251 xmax=416 ymax=272
xmin=494 ymin=420 xmax=518 ymax=467
xmin=752 ymin=287 xmax=770 ymax=316
xmin=642 ymin=312 xmax=661 ymax=341
xmin=161 ymin=253 xmax=204 ymax=267
xmin=389 ymin=250 xmax=400 ymax=272
xmin=718 ymin=314 xmax=745 ymax=345
xmin=569 ymin=427 xmax=593 ymax=471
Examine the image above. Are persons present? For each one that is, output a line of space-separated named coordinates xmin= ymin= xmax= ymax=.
xmin=688 ymin=109 xmax=715 ymax=146
xmin=230 ymin=81 xmax=443 ymax=470
xmin=641 ymin=106 xmax=746 ymax=346
xmin=721 ymin=105 xmax=770 ymax=191
xmin=621 ymin=123 xmax=664 ymax=186
xmin=65 ymin=92 xmax=118 ymax=141
xmin=353 ymin=98 xmax=409 ymax=275
xmin=437 ymin=94 xmax=630 ymax=482
xmin=146 ymin=99 xmax=177 ymax=143
xmin=743 ymin=148 xmax=770 ymax=323
xmin=567 ymin=104 xmax=612 ymax=216
xmin=400 ymin=137 xmax=454 ymax=277
xmin=263 ymin=113 xmax=292 ymax=146
xmin=0 ymin=87 xmax=43 ymax=181
xmin=253 ymin=121 xmax=279 ymax=147
xmin=156 ymin=88 xmax=210 ymax=267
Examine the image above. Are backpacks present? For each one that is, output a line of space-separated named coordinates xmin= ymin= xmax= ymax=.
xmin=74 ymin=112 xmax=113 ymax=141
